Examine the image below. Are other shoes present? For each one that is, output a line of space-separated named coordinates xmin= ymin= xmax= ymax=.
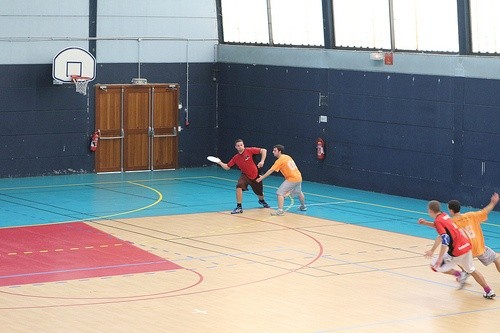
xmin=483 ymin=290 xmax=496 ymax=298
xmin=271 ymin=210 xmax=284 ymax=216
xmin=258 ymin=200 xmax=270 ymax=208
xmin=298 ymin=207 xmax=307 ymax=211
xmin=231 ymin=208 xmax=243 ymax=214
xmin=460 ymin=273 xmax=469 ymax=281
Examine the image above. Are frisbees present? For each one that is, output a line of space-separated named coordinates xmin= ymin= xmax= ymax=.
xmin=206 ymin=156 xmax=221 ymax=163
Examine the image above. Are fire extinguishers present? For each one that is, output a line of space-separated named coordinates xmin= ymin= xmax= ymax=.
xmin=317 ymin=138 xmax=326 ymax=160
xmin=90 ymin=129 xmax=101 ymax=152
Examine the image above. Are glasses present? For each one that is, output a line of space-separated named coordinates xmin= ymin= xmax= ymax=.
xmin=238 ymin=145 xmax=242 ymax=147
xmin=273 ymin=150 xmax=276 ymax=152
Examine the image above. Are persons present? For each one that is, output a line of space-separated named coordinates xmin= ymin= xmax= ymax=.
xmin=423 ymin=192 xmax=500 ymax=282
xmin=218 ymin=139 xmax=271 ymax=215
xmin=417 ymin=200 xmax=495 ymax=299
xmin=257 ymin=144 xmax=307 ymax=216
